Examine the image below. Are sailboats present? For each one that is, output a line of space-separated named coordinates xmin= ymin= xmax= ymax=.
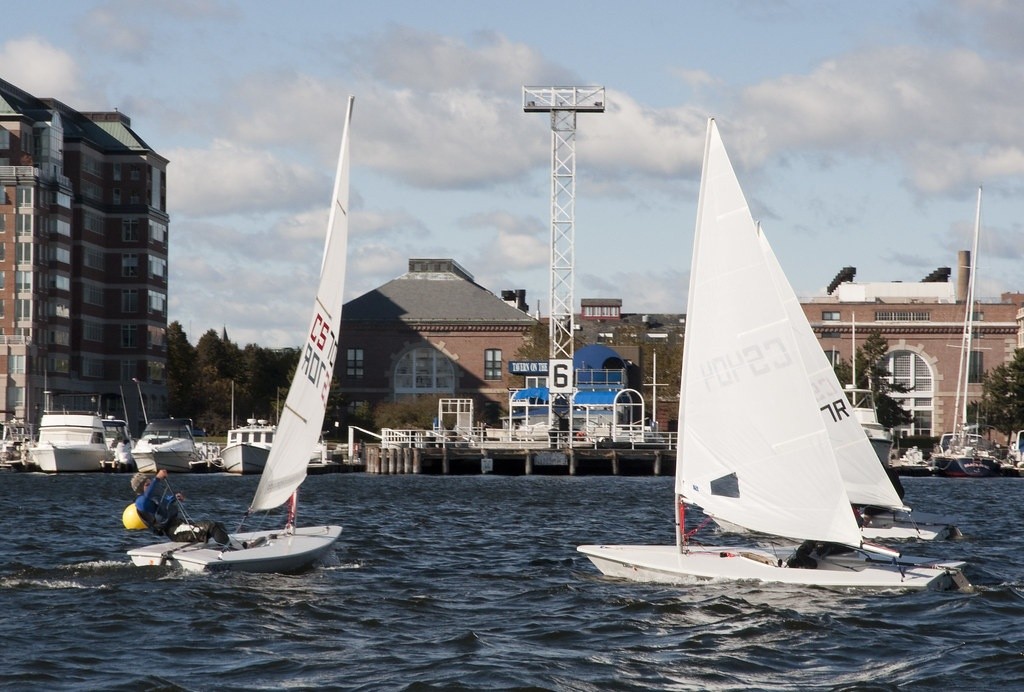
xmin=932 ymin=187 xmax=1001 ymax=476
xmin=126 ymin=96 xmax=354 ymax=575
xmin=702 ymin=221 xmax=963 ymax=544
xmin=576 ymin=115 xmax=979 ymax=594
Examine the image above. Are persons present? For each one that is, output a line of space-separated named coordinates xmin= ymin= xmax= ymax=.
xmin=130 ymin=469 xmax=247 ymax=551
xmin=862 ymin=461 xmax=905 ymax=527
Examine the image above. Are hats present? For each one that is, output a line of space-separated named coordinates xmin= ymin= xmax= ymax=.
xmin=130 ymin=473 xmax=150 ymax=493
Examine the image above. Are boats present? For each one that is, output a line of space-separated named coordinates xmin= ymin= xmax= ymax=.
xmin=30 ymin=391 xmax=138 ymax=471
xmin=842 ymin=311 xmax=894 ymax=510
xmin=220 ymin=418 xmax=278 ymax=473
xmin=130 ymin=418 xmax=200 ymax=471
